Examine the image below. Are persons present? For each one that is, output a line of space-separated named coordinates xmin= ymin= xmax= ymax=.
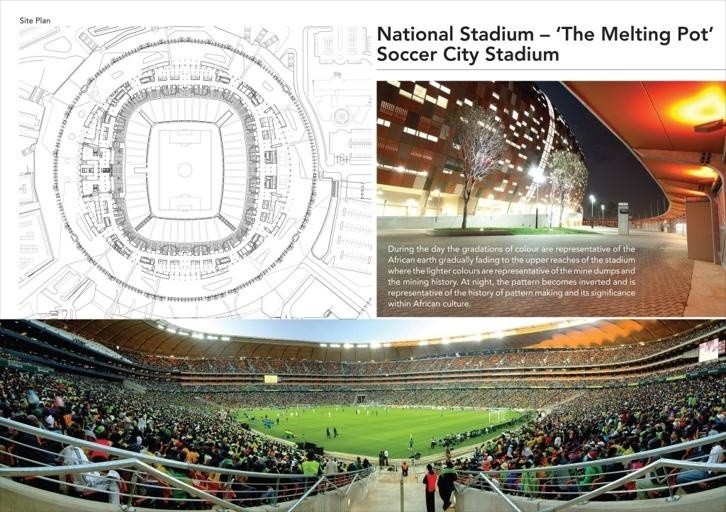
xmin=1 ymin=321 xmax=725 ymax=512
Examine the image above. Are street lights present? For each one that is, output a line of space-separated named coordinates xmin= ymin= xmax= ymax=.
xmin=590 ymin=195 xmax=596 ymax=229
xmin=601 ymin=204 xmax=605 ymax=225
xmin=529 ymin=167 xmax=546 ymax=230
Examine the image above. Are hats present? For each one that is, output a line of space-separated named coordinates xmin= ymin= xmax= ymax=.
xmin=587 ymin=449 xmax=599 ymax=459
xmin=500 ymin=462 xmax=509 ymax=470
